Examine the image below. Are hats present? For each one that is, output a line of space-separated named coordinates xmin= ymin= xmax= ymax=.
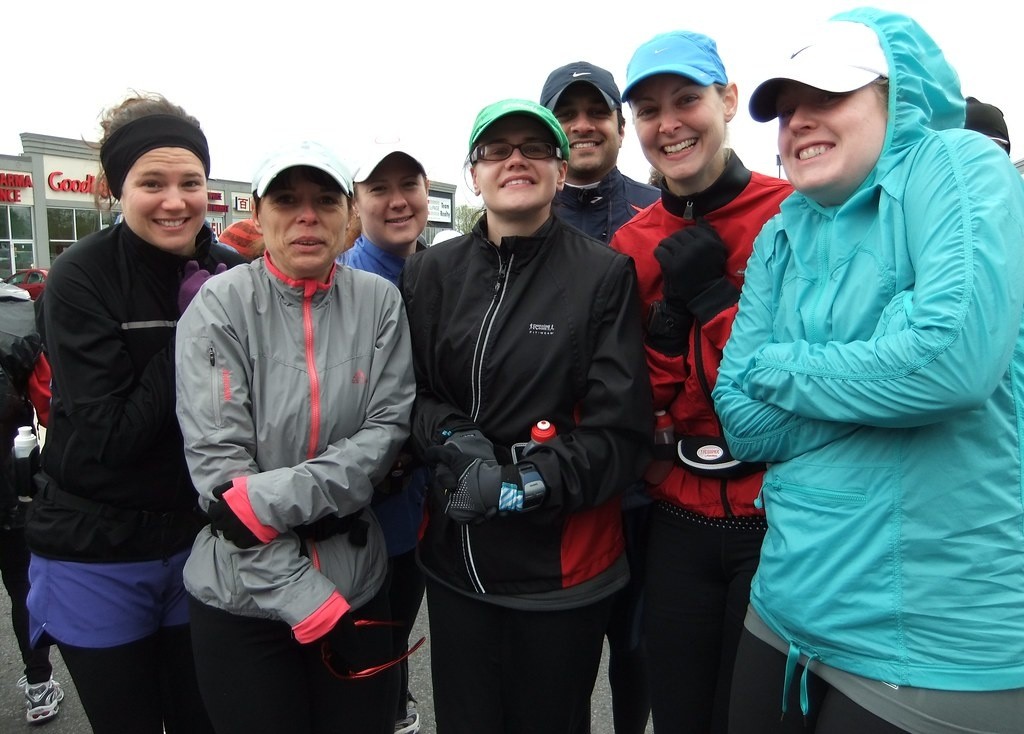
xmin=749 ymin=23 xmax=887 ymax=122
xmin=250 ymin=144 xmax=355 ymax=206
xmin=963 ymin=97 xmax=1012 ymax=156
xmin=352 ymin=143 xmax=428 ymax=199
xmin=620 ymin=30 xmax=728 ymax=103
xmin=540 ymin=61 xmax=621 ymax=121
xmin=220 ymin=220 xmax=264 ymax=260
xmin=467 ymin=100 xmax=569 ymax=165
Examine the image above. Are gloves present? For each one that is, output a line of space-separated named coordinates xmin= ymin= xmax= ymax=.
xmin=206 ymin=480 xmax=260 ymax=549
xmin=428 ymin=429 xmax=521 ymax=526
xmin=655 ymin=217 xmax=728 ymax=310
xmin=178 ymin=260 xmax=225 ymax=313
xmin=332 ymin=616 xmax=376 ymax=677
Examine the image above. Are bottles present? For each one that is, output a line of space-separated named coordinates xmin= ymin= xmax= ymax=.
xmin=655 ymin=410 xmax=675 ymax=443
xmin=522 ymin=420 xmax=560 ymax=456
xmin=14 ymin=426 xmax=37 ymax=502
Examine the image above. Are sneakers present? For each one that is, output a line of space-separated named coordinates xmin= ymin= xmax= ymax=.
xmin=16 ymin=674 xmax=64 ymax=723
xmin=394 ymin=691 xmax=420 ymax=734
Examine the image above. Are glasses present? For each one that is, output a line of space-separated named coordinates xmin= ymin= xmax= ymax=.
xmin=472 ymin=141 xmax=563 ymax=163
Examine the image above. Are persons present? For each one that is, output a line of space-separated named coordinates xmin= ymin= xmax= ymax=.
xmin=0 ymin=276 xmax=64 ymax=725
xmin=175 ymin=145 xmax=416 ymax=734
xmin=539 ymin=62 xmax=664 ymax=734
xmin=345 ymin=133 xmax=434 ymax=734
xmin=612 ymin=29 xmax=810 ymax=734
xmin=404 ymin=100 xmax=652 ymax=734
xmin=719 ymin=7 xmax=1024 ymax=733
xmin=964 ymin=96 xmax=1011 ymax=165
xmin=28 ymin=95 xmax=251 ymax=734
